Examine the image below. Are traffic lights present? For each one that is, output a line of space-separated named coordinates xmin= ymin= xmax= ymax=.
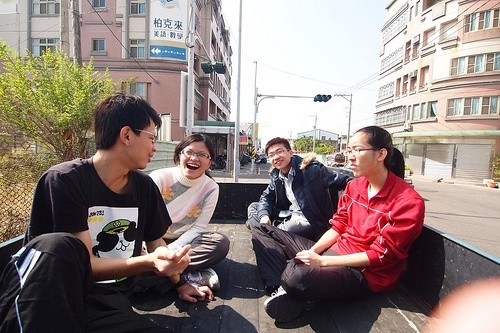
xmin=200 ymin=62 xmax=213 ymax=74
xmin=214 ymin=63 xmax=225 ymax=74
xmin=314 ymin=94 xmax=332 ymax=102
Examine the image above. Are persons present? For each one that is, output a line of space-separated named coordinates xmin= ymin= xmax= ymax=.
xmin=251 ymin=126 xmax=425 ymax=323
xmin=140 ymin=133 xmax=230 ymax=290
xmin=0 ymin=94 xmax=215 ymax=333
xmin=246 ymin=136 xmax=355 ymax=250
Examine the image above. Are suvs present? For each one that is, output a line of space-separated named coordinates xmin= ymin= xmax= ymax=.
xmin=257 ymin=153 xmax=267 ymax=164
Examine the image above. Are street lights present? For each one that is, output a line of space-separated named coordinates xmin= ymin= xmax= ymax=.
xmin=252 ymin=97 xmax=275 ymax=171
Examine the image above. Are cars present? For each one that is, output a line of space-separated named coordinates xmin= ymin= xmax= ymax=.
xmin=325 ymin=160 xmax=414 ymax=194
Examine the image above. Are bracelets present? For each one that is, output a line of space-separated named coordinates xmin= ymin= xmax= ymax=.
xmin=172 ymin=279 xmax=185 ymax=288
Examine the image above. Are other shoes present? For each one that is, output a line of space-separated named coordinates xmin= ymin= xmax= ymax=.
xmin=263 ymin=285 xmax=317 ymax=323
xmin=185 ymin=268 xmax=221 ymax=292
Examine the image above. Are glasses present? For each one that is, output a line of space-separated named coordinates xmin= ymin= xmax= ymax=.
xmin=130 ymin=128 xmax=157 ymax=143
xmin=343 ymin=148 xmax=375 ymax=157
xmin=180 ymin=149 xmax=212 ymax=162
xmin=266 ymin=149 xmax=289 ymax=161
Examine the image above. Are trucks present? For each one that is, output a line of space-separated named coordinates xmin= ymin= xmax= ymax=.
xmin=326 ymin=153 xmax=345 ymax=167
